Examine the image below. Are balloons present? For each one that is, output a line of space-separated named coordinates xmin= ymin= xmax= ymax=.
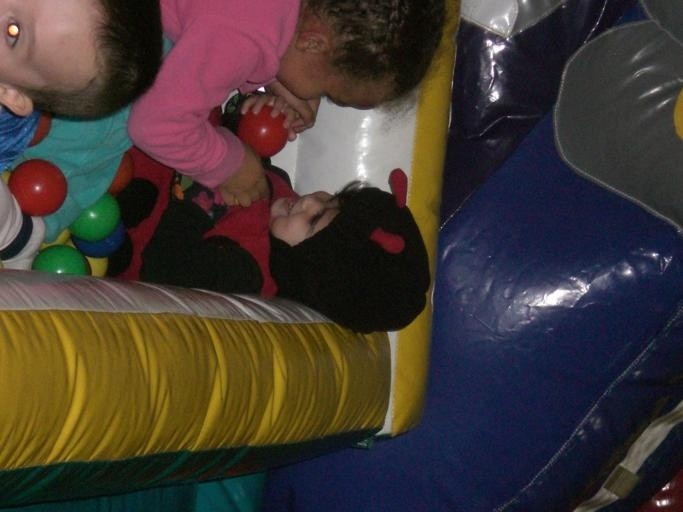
xmin=9 ymin=158 xmax=124 ymax=276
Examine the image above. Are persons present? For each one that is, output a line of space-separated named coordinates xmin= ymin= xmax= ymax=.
xmin=0 ymin=0 xmax=163 ymax=271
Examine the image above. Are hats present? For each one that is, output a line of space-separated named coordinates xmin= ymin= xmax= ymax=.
xmin=267 ymin=186 xmax=431 ymax=334
